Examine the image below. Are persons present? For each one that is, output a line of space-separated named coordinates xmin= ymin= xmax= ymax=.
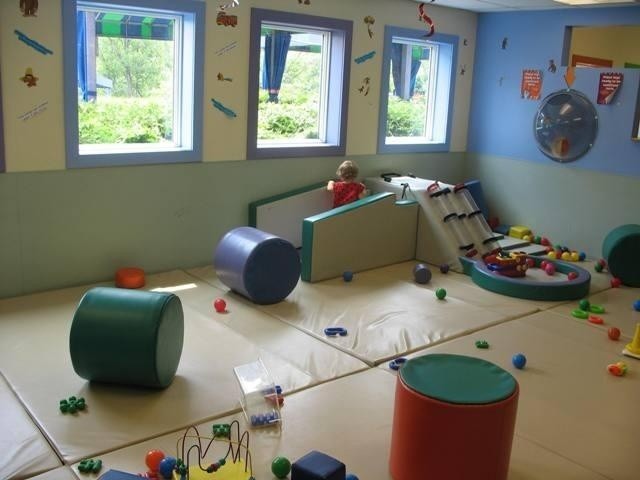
xmin=327 ymin=160 xmax=367 ymax=208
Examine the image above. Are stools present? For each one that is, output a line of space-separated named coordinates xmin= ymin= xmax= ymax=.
xmin=386 ymin=351 xmax=518 ymax=480
xmin=602 ymin=222 xmax=640 ymax=287
xmin=214 ymin=226 xmax=301 ymax=306
xmin=68 ymin=285 xmax=186 ymax=392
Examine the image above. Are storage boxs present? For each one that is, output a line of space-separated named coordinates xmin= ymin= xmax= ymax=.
xmin=233 ymin=355 xmax=284 ymax=429
